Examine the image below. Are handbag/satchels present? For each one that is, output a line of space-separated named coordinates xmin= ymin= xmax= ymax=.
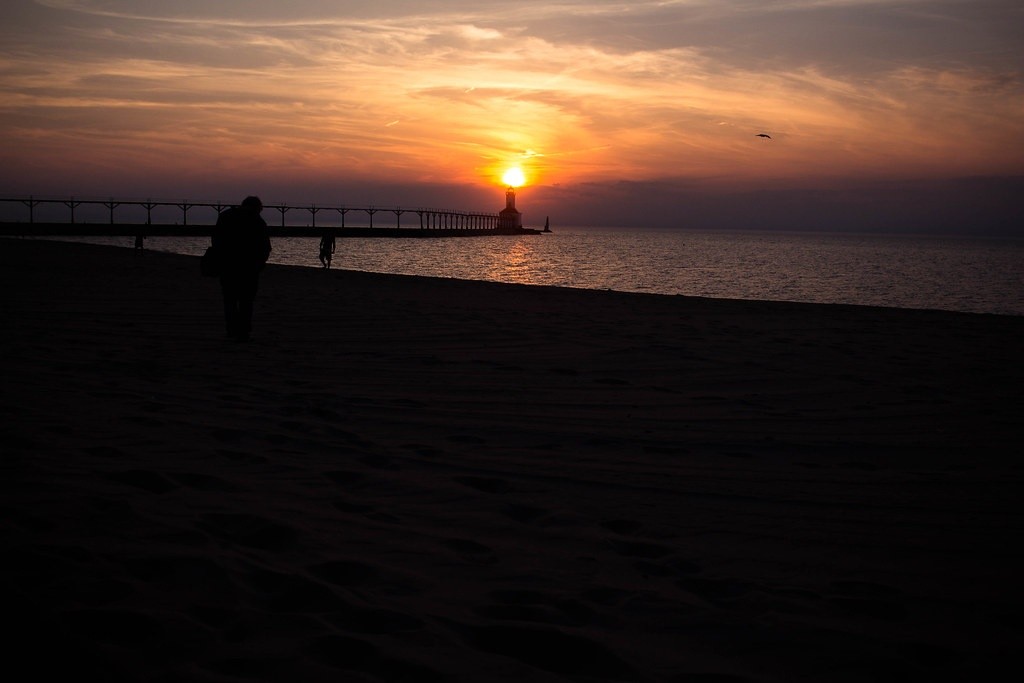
xmin=199 ymin=247 xmax=224 ymax=277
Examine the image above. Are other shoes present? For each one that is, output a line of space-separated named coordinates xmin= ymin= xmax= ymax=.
xmin=226 ymin=330 xmax=238 ymax=338
xmin=236 ymin=335 xmax=256 ymax=342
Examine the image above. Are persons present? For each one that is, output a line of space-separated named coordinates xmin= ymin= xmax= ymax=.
xmin=134 ymin=228 xmax=146 ymax=254
xmin=211 ymin=197 xmax=272 ymax=341
xmin=319 ymin=228 xmax=336 ymax=269
